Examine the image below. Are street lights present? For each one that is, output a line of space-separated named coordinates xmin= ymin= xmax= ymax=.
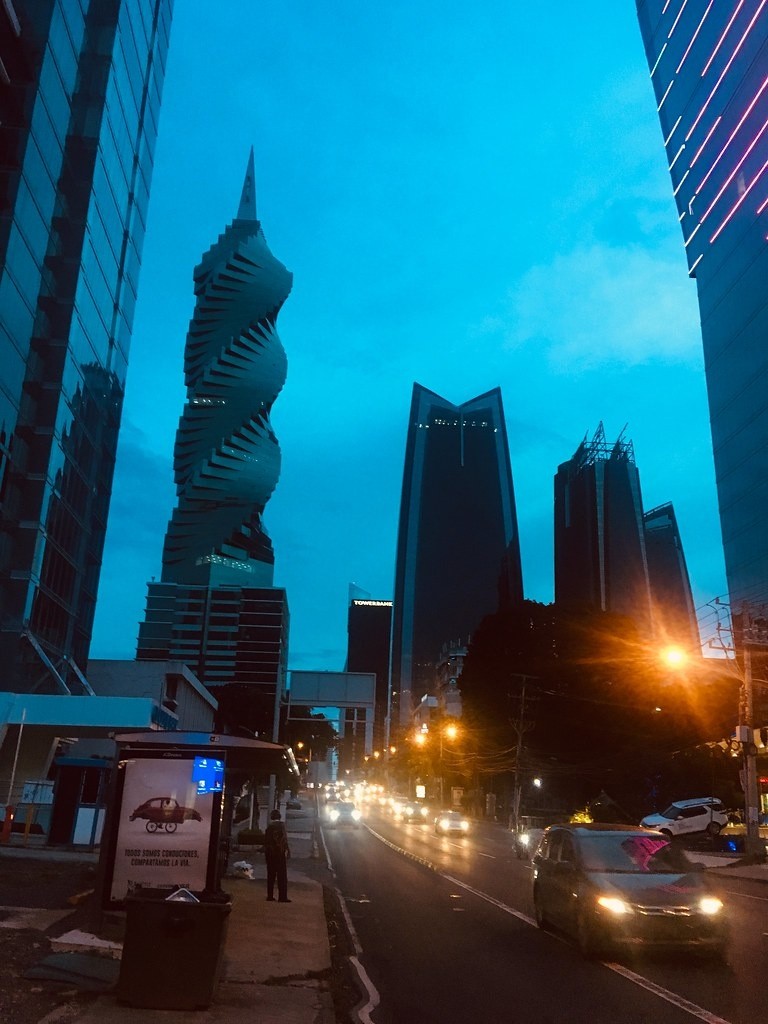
xmin=667 ymin=648 xmax=760 ymax=855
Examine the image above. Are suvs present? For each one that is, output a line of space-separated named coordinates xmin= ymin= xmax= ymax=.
xmin=530 ymin=823 xmax=728 ymax=964
xmin=325 ymin=801 xmax=360 ymax=829
xmin=639 ymin=797 xmax=729 ymax=838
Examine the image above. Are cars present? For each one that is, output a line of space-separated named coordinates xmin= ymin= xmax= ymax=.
xmin=434 ymin=810 xmax=467 ymax=836
xmin=403 ymin=803 xmax=427 ymax=823
xmin=370 ymin=784 xmax=409 ymax=816
xmin=365 ymin=794 xmax=376 ymax=806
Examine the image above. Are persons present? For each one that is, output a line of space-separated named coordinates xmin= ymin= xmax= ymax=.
xmin=262 ymin=810 xmax=292 ymax=902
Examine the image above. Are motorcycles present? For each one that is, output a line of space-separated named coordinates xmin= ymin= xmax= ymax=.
xmin=512 ymin=829 xmax=530 ymax=859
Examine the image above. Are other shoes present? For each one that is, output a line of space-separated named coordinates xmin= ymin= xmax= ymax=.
xmin=266 ymin=897 xmax=276 ymax=902
xmin=278 ymin=899 xmax=291 ymax=902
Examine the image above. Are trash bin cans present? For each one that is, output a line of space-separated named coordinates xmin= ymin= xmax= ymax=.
xmin=116 ymin=888 xmax=234 ymax=1011
